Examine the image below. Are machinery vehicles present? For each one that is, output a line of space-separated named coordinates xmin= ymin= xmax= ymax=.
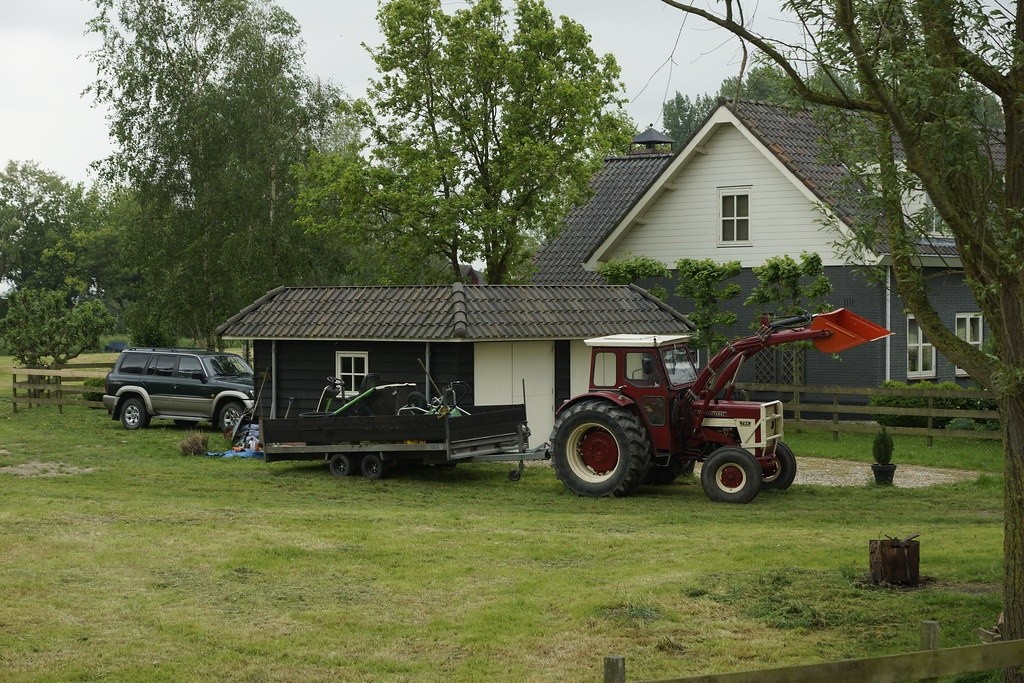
xmin=549 ymin=306 xmax=896 ymax=504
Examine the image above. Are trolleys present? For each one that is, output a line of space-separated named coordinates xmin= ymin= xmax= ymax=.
xmin=397 ymin=388 xmax=471 ymax=416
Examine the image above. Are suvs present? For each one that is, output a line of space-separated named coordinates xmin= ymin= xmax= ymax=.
xmin=102 ymin=347 xmax=255 ymax=430
